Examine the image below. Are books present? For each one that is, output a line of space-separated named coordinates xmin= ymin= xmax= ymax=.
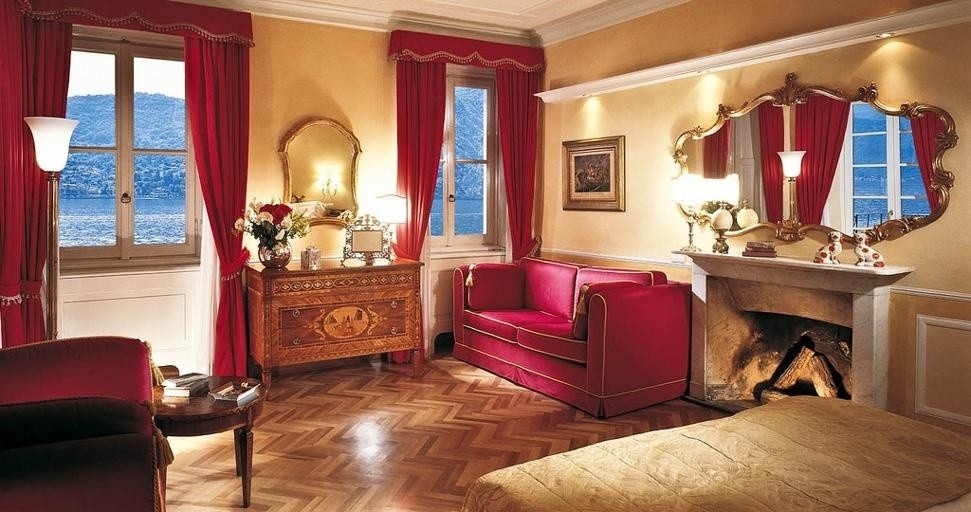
xmin=163 ymin=379 xmax=209 ymax=397
xmin=161 ymin=373 xmax=207 ymax=387
xmin=742 ymin=241 xmax=778 ymax=258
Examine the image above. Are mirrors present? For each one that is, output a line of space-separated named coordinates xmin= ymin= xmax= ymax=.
xmin=665 ymin=74 xmax=963 ymax=245
xmin=279 ymin=119 xmax=362 ymax=228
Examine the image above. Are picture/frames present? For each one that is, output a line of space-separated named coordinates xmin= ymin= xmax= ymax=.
xmin=562 ymin=135 xmax=625 ymax=213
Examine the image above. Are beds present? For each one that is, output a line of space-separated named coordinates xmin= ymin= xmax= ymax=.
xmin=463 ymin=393 xmax=970 ymax=512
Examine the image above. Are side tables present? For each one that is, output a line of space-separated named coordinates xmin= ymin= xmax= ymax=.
xmin=154 ymin=375 xmax=267 ymax=506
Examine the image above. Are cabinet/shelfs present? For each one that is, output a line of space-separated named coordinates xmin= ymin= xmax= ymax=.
xmin=245 ymin=259 xmax=425 ymax=389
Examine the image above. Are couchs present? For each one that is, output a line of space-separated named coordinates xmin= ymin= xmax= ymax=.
xmin=452 ymin=256 xmax=692 ymax=418
xmin=0 ymin=336 xmax=174 ymax=512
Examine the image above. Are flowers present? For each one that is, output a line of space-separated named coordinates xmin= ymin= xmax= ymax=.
xmin=231 ymin=196 xmax=312 ymax=239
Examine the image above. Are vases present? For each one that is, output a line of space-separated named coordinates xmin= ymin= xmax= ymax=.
xmin=258 ymin=240 xmax=291 ymax=268
xmin=256 ymin=240 xmax=296 ymax=269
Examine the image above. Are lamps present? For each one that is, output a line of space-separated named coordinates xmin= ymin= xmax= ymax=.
xmin=777 ymin=150 xmax=805 ymax=243
xmin=24 ymin=117 xmax=79 ymax=339
xmin=374 ymin=193 xmax=409 ymax=261
xmin=707 ymin=174 xmax=740 ymax=255
xmin=672 ymin=173 xmax=708 ymax=254
xmin=312 ymin=158 xmax=341 ymax=209
